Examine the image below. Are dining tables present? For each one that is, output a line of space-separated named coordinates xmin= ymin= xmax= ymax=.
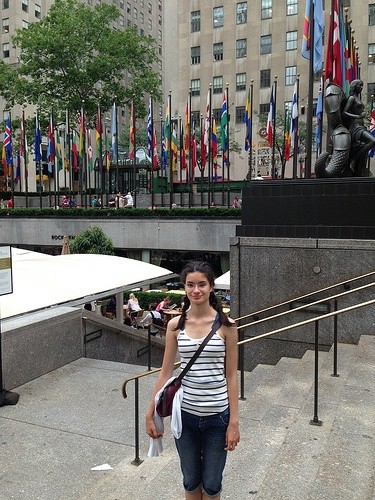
xmin=163 ymin=308 xmax=182 ymax=318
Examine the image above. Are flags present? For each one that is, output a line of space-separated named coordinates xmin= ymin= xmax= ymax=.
xmin=316 ymin=75 xmax=323 ymax=148
xmin=367 ymin=109 xmax=375 ymax=157
xmin=244 ymin=85 xmax=253 ymax=151
xmin=266 ymin=82 xmax=276 ymax=149
xmin=1 ymin=87 xmax=231 ymax=184
xmin=301 ymin=0 xmax=325 ymax=74
xmin=290 ymin=80 xmax=300 ymax=156
xmin=283 ymin=110 xmax=290 ymax=161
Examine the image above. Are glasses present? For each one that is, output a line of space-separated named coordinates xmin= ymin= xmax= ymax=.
xmin=185 ymin=282 xmax=209 ymax=288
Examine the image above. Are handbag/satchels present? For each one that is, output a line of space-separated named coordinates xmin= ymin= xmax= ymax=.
xmin=156 ymin=378 xmax=182 ymax=417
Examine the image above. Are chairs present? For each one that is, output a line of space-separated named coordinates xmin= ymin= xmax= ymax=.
xmin=130 ymin=309 xmax=144 ymax=330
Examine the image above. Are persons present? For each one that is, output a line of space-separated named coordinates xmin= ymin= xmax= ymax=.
xmin=0 ymin=198 xmax=13 ymax=209
xmin=341 ymin=79 xmax=375 ymax=173
xmin=127 ymin=292 xmax=143 ymax=320
xmin=60 ymin=193 xmax=77 ymax=208
xmin=82 ymin=300 xmax=97 ymax=313
xmin=104 ymin=299 xmax=116 ymax=319
xmin=140 ymin=305 xmax=162 ymax=336
xmin=154 ymin=295 xmax=185 ymax=321
xmin=91 ymin=194 xmax=102 ymax=208
xmin=146 ymin=260 xmax=240 ymax=500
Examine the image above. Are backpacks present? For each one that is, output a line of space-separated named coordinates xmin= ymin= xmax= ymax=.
xmin=150 ymin=312 xmax=164 ymax=331
